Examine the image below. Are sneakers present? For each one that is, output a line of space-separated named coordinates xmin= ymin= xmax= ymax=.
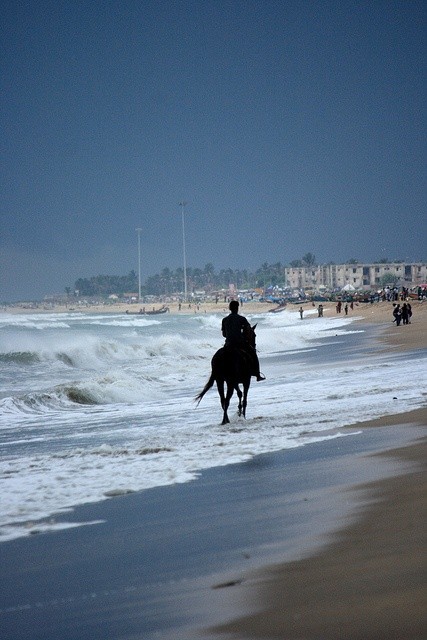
xmin=257 ymin=377 xmax=266 ymax=381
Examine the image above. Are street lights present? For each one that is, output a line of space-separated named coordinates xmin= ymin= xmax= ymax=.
xmin=135 ymin=226 xmax=144 ymax=301
xmin=176 ymin=198 xmax=190 ymax=303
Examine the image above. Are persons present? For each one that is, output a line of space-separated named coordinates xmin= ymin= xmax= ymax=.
xmin=188 ymin=300 xmax=200 ymax=314
xmin=179 ymin=301 xmax=183 ymax=311
xmin=381 ymin=286 xmax=416 ymax=302
xmin=271 ymin=293 xmax=327 ymax=306
xmin=418 ymin=286 xmax=427 ymax=301
xmin=317 ymin=305 xmax=324 ymax=317
xmin=335 ymin=301 xmax=353 ymax=315
xmin=222 ymin=301 xmax=266 ymax=381
xmin=392 ymin=302 xmax=412 ymax=326
xmin=299 ymin=307 xmax=303 ymax=319
xmin=332 ymin=290 xmax=378 ymax=301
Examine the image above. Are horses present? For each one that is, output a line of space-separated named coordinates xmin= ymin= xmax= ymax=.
xmin=192 ymin=322 xmax=259 ymax=427
